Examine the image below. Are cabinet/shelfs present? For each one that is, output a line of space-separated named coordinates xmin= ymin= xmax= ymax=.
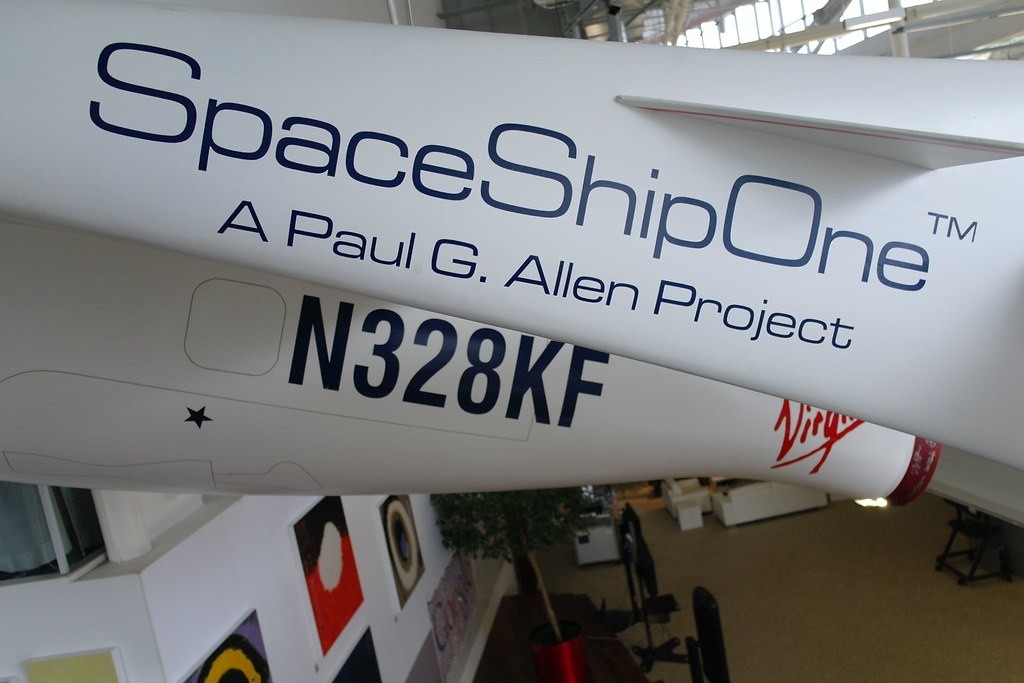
xmin=574 ymin=513 xmax=620 ymax=565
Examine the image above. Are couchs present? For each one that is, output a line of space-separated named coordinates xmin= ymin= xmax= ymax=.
xmin=712 ymin=482 xmax=828 ymax=527
xmin=661 ymin=479 xmax=713 ymax=519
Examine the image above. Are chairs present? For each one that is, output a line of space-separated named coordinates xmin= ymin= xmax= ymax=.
xmin=934 ymin=498 xmax=1013 ymax=586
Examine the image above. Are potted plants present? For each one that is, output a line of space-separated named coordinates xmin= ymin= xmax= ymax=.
xmin=430 ymin=485 xmax=610 ymax=683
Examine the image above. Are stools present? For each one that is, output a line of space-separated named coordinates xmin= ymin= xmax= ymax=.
xmin=675 ymin=501 xmax=704 ymax=531
